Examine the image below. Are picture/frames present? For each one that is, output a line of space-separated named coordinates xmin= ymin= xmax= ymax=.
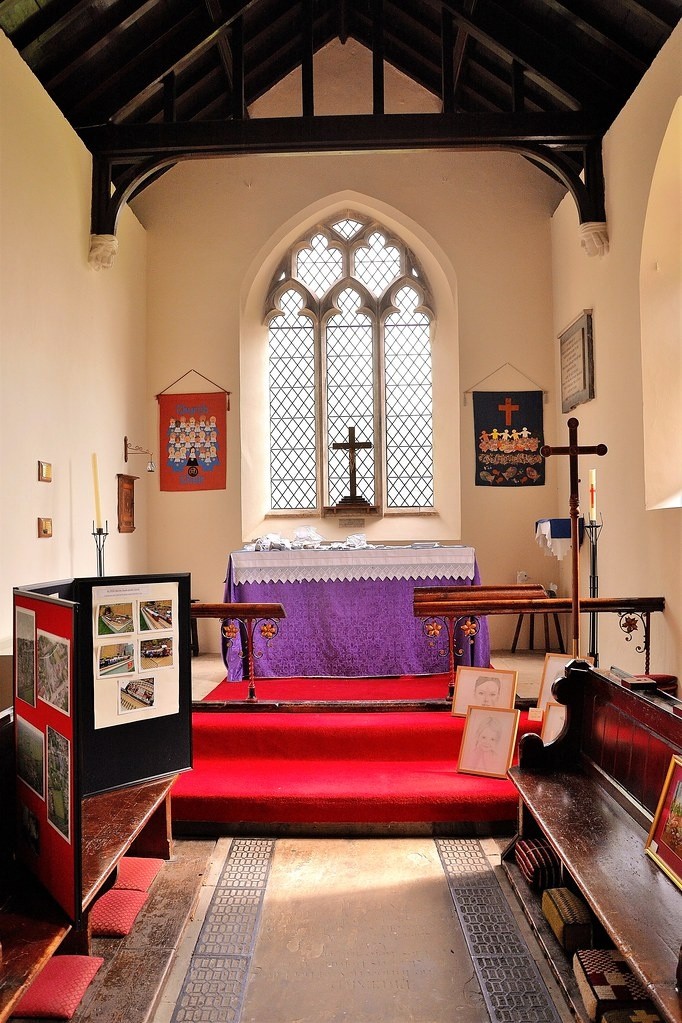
xmin=451 ymin=654 xmax=595 ymax=780
xmin=644 ymin=753 xmax=682 ymax=893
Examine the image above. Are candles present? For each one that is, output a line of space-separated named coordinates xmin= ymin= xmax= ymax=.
xmin=589 ymin=468 xmax=597 ymax=520
xmin=93 ymin=453 xmax=102 ymax=529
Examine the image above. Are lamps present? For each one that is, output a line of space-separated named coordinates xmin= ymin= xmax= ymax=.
xmin=124 ymin=436 xmax=157 ymax=473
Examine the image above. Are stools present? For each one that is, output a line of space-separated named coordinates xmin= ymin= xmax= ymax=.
xmin=510 ymin=589 xmax=566 ymax=655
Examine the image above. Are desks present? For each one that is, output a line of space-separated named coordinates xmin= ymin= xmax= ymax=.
xmin=221 ymin=546 xmax=492 ymax=679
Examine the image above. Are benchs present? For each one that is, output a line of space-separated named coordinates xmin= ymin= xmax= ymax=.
xmin=0 ymin=721 xmax=180 ymax=1023
xmin=505 ymin=656 xmax=682 ymax=1023
